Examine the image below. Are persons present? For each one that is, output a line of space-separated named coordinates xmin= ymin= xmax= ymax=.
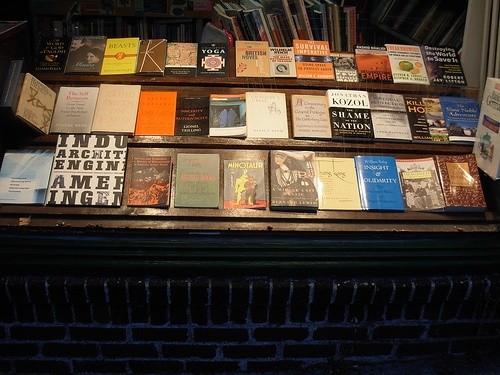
xmin=67 ymin=37 xmax=88 ymax=67
xmin=86 ymin=48 xmax=102 ymax=71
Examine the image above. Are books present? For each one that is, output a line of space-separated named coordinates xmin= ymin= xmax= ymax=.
xmin=0 ymin=1 xmax=500 ymax=213
